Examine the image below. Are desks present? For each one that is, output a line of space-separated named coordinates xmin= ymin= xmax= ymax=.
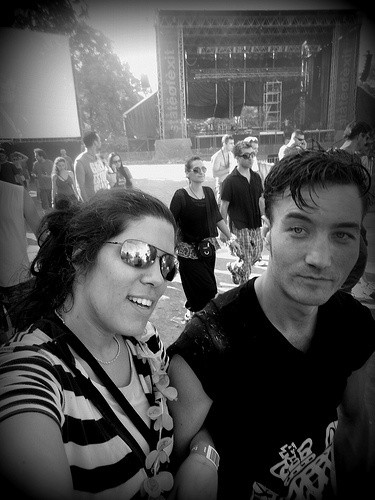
xmin=260 ymin=131 xmax=285 ymax=144
xmin=198 ymin=134 xmax=231 ymax=150
xmin=304 ymin=130 xmax=336 ymax=147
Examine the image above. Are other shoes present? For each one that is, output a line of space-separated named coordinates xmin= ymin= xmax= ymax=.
xmin=226 ymin=262 xmax=239 ymax=285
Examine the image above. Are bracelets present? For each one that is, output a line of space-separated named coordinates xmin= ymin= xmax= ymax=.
xmin=42 ymin=209 xmax=45 ymax=216
xmin=50 ymin=201 xmax=54 ymax=204
xmin=190 ymin=442 xmax=220 ymax=469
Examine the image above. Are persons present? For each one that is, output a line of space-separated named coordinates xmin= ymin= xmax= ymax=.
xmin=338 ymin=121 xmax=375 ymax=179
xmin=49 ymin=156 xmax=81 ymax=209
xmin=278 ymin=129 xmax=308 ymax=161
xmin=0 ymin=178 xmax=51 ymax=349
xmin=0 ymin=186 xmax=220 ymax=500
xmin=30 ymin=148 xmax=54 ymax=209
xmin=74 ymin=130 xmax=115 ymax=206
xmin=164 ymin=149 xmax=375 ymax=500
xmin=0 ymin=141 xmax=30 ymax=218
xmin=210 ymin=134 xmax=238 ymax=244
xmin=171 ymin=156 xmax=242 ymax=324
xmin=219 ymin=140 xmax=266 ymax=285
xmin=126 ymin=251 xmax=150 ymax=267
xmin=108 ymin=150 xmax=139 ymax=192
xmin=245 ymin=136 xmax=272 ymax=238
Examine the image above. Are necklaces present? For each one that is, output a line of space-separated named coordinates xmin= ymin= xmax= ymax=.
xmin=54 ymin=309 xmax=121 ymax=365
xmin=189 ymin=185 xmax=203 ymax=200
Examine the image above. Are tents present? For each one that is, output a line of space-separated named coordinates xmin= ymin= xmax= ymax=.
xmin=122 ymin=89 xmax=158 ymax=152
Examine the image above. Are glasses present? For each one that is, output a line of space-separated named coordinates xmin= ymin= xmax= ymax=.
xmin=112 ymin=160 xmax=121 ymax=164
xmin=105 ymin=239 xmax=179 ymax=282
xmin=239 ymin=151 xmax=256 ymax=159
xmin=296 ymin=137 xmax=305 ymax=141
xmin=188 ymin=166 xmax=207 ymax=173
